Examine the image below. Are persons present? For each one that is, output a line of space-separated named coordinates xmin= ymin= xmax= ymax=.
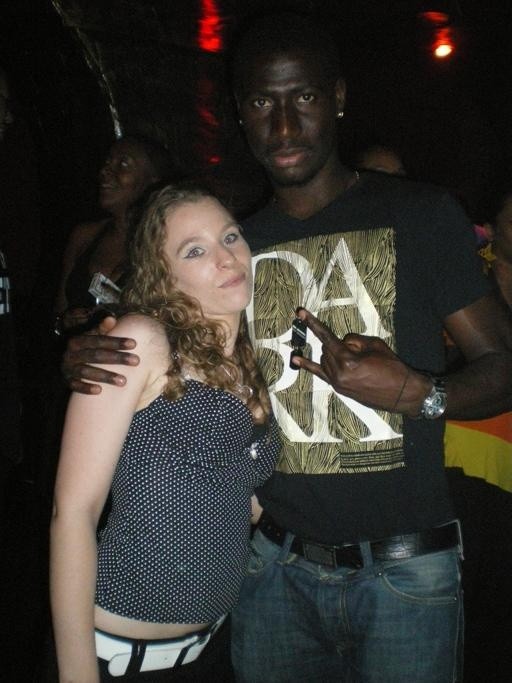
xmin=48 ymin=38 xmax=511 ymax=683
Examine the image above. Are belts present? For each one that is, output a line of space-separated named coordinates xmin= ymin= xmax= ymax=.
xmin=96 ymin=613 xmax=228 ymax=678
xmin=257 ymin=510 xmax=460 ymax=570
